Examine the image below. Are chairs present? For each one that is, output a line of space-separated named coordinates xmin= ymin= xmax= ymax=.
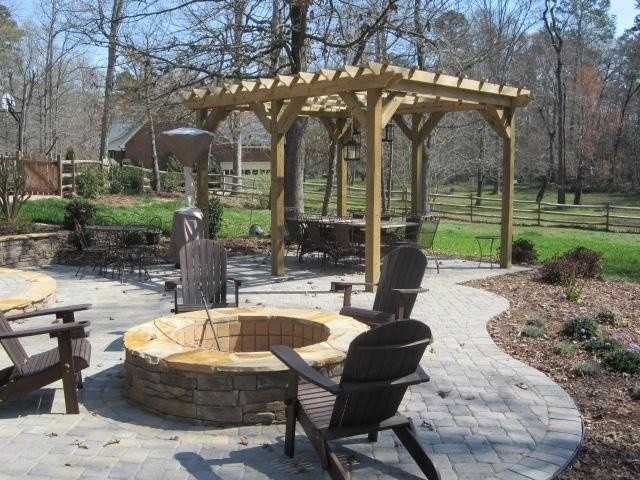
xmin=330 ymin=246 xmax=427 ymax=329
xmin=269 ymin=319 xmax=442 ymax=479
xmin=284 ymin=206 xmax=441 ymax=274
xmin=165 ymin=239 xmax=248 ymax=314
xmin=0 ymin=303 xmax=92 ymax=414
xmin=73 ymin=215 xmax=163 ymax=285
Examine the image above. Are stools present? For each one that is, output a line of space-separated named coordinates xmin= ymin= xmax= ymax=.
xmin=475 ymin=235 xmax=501 ymax=269
